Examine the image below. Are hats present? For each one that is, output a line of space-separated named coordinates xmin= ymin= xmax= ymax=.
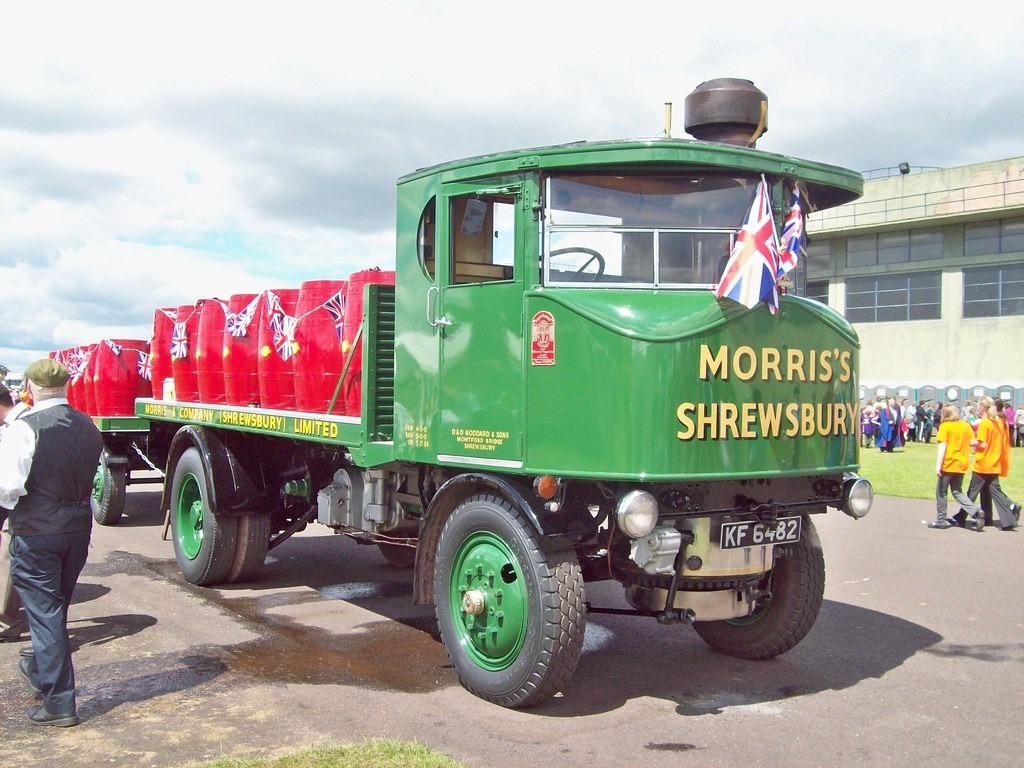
xmin=24 ymin=358 xmax=70 ymax=387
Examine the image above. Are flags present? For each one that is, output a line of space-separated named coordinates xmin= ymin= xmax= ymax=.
xmin=323 ymin=293 xmax=344 ymax=350
xmin=170 ymin=325 xmax=187 ymax=358
xmin=225 ymin=297 xmax=259 ymax=336
xmin=715 ymin=179 xmax=779 ymax=317
xmin=137 ymin=354 xmax=151 ymax=381
xmin=776 ymin=185 xmax=803 ymax=280
xmin=266 ymin=291 xmax=297 ymax=361
xmin=105 ymin=340 xmax=122 ymax=355
xmin=67 ymin=350 xmax=90 ymax=386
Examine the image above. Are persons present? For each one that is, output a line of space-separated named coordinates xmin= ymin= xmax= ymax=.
xmin=860 ymin=396 xmax=943 ymax=453
xmin=946 ymin=396 xmax=1021 ymax=531
xmin=959 ymin=400 xmax=1024 ymax=448
xmin=0 ymin=359 xmax=103 ymax=728
xmin=927 ymin=405 xmax=985 ymax=533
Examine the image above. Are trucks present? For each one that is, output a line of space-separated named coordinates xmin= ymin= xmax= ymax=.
xmin=133 ymin=78 xmax=875 ymax=711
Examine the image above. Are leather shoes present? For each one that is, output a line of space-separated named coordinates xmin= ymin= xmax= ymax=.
xmin=25 ymin=704 xmax=78 ymax=727
xmin=18 ymin=657 xmax=43 ymax=694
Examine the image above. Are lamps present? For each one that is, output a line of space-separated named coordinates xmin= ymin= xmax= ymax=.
xmin=899 ymin=162 xmax=911 ymax=174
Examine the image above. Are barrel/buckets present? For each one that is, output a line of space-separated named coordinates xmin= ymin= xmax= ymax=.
xmin=48 ymin=270 xmax=396 ymax=418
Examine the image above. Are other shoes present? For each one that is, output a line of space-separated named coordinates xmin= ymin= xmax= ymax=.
xmin=975 ymin=510 xmax=985 ymax=531
xmin=928 ymin=522 xmax=946 ymax=529
xmin=0 ymin=621 xmax=22 ymax=643
xmin=946 ymin=518 xmax=966 ymax=527
xmin=1012 ymin=505 xmax=1021 ymax=521
